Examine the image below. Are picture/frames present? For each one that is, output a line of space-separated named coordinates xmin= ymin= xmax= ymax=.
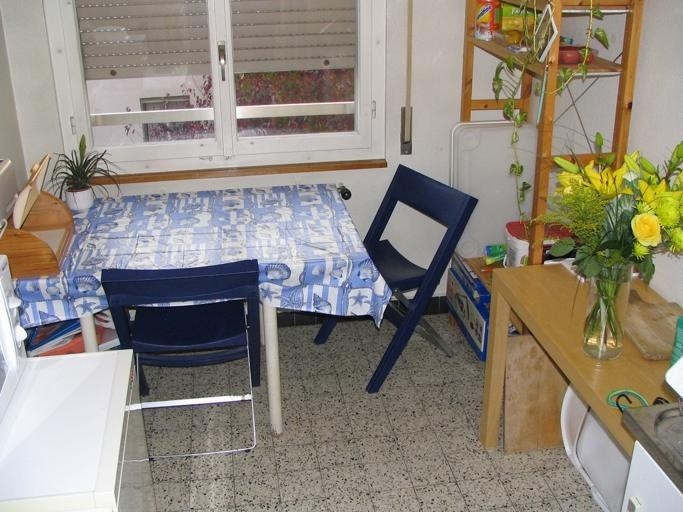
xmin=534 ymin=4 xmax=560 ymax=63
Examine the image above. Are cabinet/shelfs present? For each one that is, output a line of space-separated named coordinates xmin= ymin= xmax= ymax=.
xmin=463 ymin=0 xmax=645 ymax=265
xmin=0 ymin=348 xmax=161 ymax=512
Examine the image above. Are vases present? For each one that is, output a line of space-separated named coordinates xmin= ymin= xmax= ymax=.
xmin=578 ymin=262 xmax=635 ymax=363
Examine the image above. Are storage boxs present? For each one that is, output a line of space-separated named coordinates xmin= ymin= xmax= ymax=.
xmin=500 ymin=220 xmax=582 ymax=269
xmin=443 ymin=267 xmax=518 ymax=362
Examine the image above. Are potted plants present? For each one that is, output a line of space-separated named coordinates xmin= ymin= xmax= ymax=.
xmin=44 ymin=134 xmax=124 ymax=212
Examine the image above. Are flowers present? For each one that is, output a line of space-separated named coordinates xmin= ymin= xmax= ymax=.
xmin=529 ymin=139 xmax=683 ymax=350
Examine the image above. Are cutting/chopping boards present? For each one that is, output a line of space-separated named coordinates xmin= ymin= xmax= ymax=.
xmin=622 ymin=290 xmax=683 ymax=362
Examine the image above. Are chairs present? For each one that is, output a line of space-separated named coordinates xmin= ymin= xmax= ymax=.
xmin=100 ymin=258 xmax=263 ymax=397
xmin=310 ymin=164 xmax=480 ymax=394
xmin=560 ymin=381 xmax=630 ymax=512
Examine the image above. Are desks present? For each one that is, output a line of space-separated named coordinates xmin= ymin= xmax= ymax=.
xmin=13 ymin=182 xmax=394 ymax=437
xmin=617 ymin=401 xmax=683 ymax=512
xmin=479 ymin=264 xmax=682 ymax=462
